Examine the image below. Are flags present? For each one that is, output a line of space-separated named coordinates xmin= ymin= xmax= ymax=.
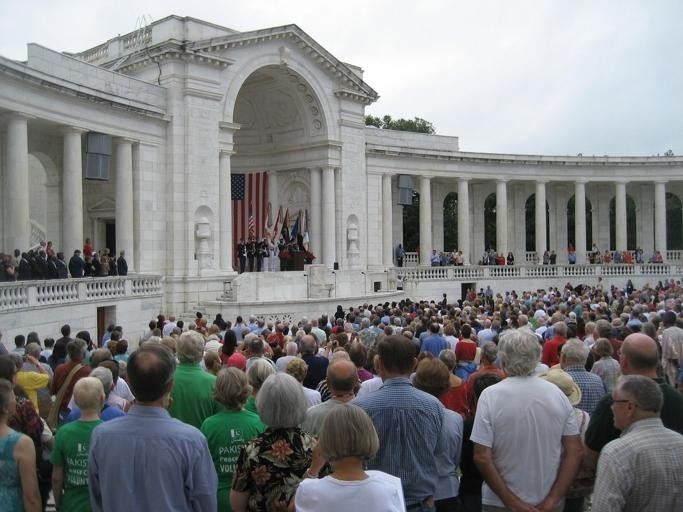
xmin=291 ymin=209 xmax=309 ymax=245
xmin=229 ymin=169 xmax=271 ymax=274
xmin=274 ymin=206 xmax=292 ymax=242
xmin=248 ymin=199 xmax=255 ymax=233
xmin=264 ymin=202 xmax=274 ymax=239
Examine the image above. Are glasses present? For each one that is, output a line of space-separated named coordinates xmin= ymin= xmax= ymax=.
xmin=609 ymin=397 xmax=630 ymax=404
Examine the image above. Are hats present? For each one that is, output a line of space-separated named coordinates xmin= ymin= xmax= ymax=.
xmin=612 ymin=318 xmax=624 ymax=328
xmin=536 ymin=369 xmax=582 ymax=406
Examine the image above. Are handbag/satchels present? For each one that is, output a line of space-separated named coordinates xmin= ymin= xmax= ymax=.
xmin=47 ymin=363 xmax=87 ymax=433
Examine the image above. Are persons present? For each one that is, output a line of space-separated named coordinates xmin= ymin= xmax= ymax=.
xmin=568 ymin=244 xmax=576 ymax=264
xmin=529 ymin=279 xmax=682 ymax=377
xmin=583 ymin=334 xmax=682 ymax=465
xmin=589 ymin=244 xmax=644 ymax=263
xmin=51 ymin=340 xmax=95 ymax=420
xmin=469 ymin=332 xmax=584 ymax=512
xmin=22 ymin=344 xmax=54 ymax=418
xmin=464 ymin=341 xmax=508 ymax=418
xmin=83 ymin=239 xmax=92 ymax=260
xmin=84 ymin=248 xmax=116 ymax=277
xmin=168 ymin=330 xmax=223 ymax=428
xmin=481 ymin=247 xmax=505 ymax=265
xmin=68 ymin=250 xmax=90 ymax=278
xmin=237 ymin=239 xmax=246 ymax=274
xmin=256 ymin=239 xmax=266 ymax=272
xmin=139 ymin=314 xmax=183 ymax=352
xmin=246 ymin=237 xmax=256 ymax=272
xmin=87 ymin=344 xmax=219 ymax=511
xmin=278 ymin=237 xmax=287 ymax=271
xmin=409 ymin=358 xmax=463 ymax=512
xmin=0 ymin=324 xmax=94 ymax=365
xmin=648 ymin=251 xmax=663 ymax=264
xmin=473 ymin=373 xmax=503 ymax=409
xmin=299 ymin=335 xmax=331 ymax=389
xmin=0 ymin=378 xmax=43 ymax=512
xmin=539 ymin=369 xmax=590 ymax=453
xmin=92 ymin=322 xmax=134 ymax=399
xmin=262 ymin=314 xmax=329 ymax=373
xmin=300 ymin=359 xmax=358 ymax=440
xmin=413 ymin=286 xmax=530 ymax=375
xmin=561 ymin=337 xmax=606 ymax=417
xmin=343 ymin=336 xmax=446 ymax=512
xmin=117 ymin=250 xmax=127 ymax=275
xmin=587 ymin=376 xmax=683 ymax=512
xmin=55 ymin=252 xmax=68 ymax=278
xmin=49 ymin=376 xmax=106 ymax=512
xmin=63 ymin=366 xmax=127 ymax=426
xmin=9 ymin=352 xmax=55 ymax=452
xmin=434 ymin=349 xmax=467 ymax=421
xmin=182 ymin=311 xmax=233 ymax=339
xmin=506 ymin=251 xmax=514 ymax=265
xmin=543 ymin=251 xmax=550 ymax=263
xmin=286 ymin=404 xmax=404 ymax=511
xmin=396 ymin=274 xmax=406 ymax=290
xmin=205 ymin=319 xmax=266 ymax=372
xmin=0 ymin=241 xmax=59 ymax=283
xmin=396 ymin=244 xmax=404 ymax=266
xmin=228 ymin=373 xmax=333 ymax=512
xmin=549 ymin=250 xmax=555 ymax=263
xmin=0 ymin=354 xmax=44 ymax=474
xmin=431 ymin=248 xmax=463 ymax=266
xmin=200 ymin=367 xmax=267 ymax=512
xmin=327 ymin=297 xmax=419 ymax=378
